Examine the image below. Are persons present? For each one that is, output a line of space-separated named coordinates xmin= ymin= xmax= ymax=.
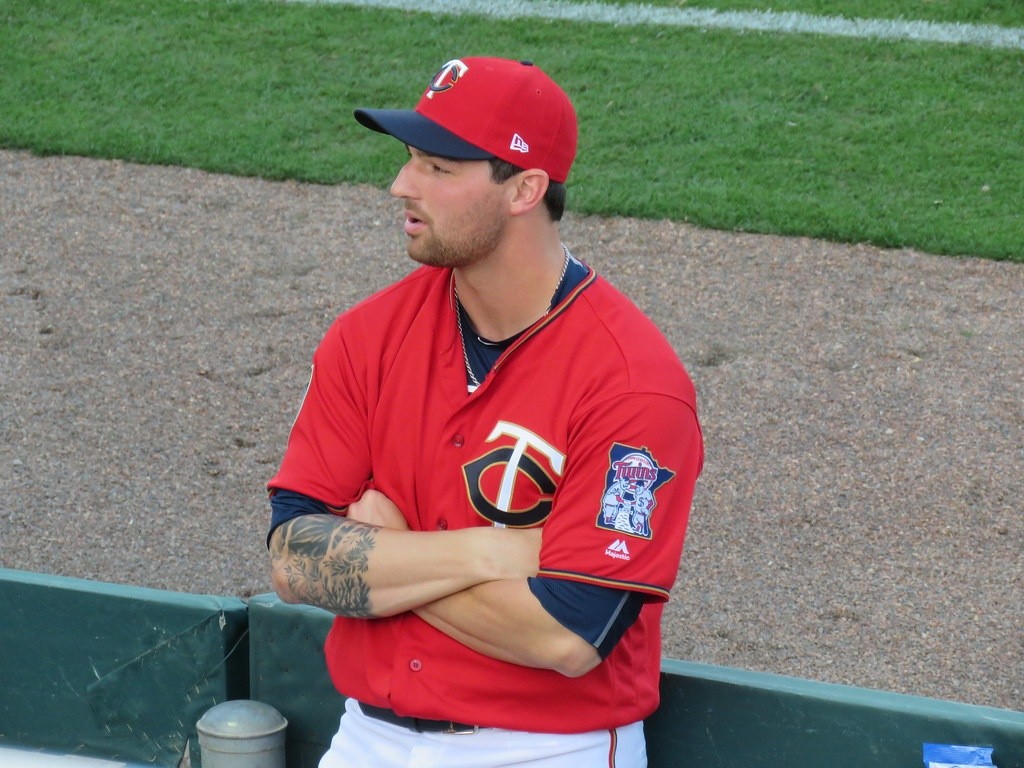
xmin=266 ymin=57 xmax=704 ymax=768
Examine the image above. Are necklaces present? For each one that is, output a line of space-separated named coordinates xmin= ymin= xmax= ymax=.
xmin=448 ymin=242 xmax=569 ymax=387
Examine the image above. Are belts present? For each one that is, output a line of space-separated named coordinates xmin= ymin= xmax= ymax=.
xmin=358 ymin=701 xmax=489 ymax=735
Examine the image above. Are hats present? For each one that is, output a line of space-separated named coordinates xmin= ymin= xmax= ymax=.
xmin=354 ymin=56 xmax=577 ymax=184
